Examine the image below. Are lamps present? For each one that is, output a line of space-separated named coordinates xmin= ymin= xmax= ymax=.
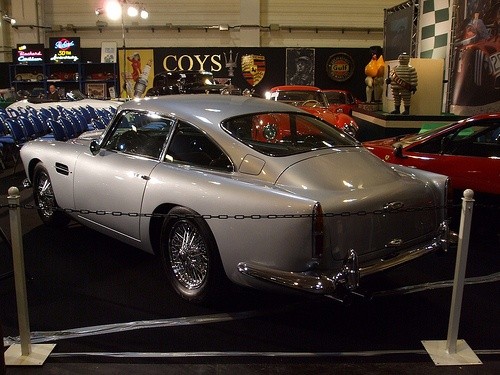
xmin=95 ymin=8 xmax=105 ymax=15
xmin=1 ymin=8 xmax=16 ymax=24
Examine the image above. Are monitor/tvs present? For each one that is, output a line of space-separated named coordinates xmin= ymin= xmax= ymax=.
xmin=49 ymin=36 xmax=80 ymax=62
xmin=17 ymin=44 xmax=44 ymax=63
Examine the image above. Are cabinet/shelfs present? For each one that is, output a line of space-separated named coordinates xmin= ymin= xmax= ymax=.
xmin=9 ymin=62 xmax=119 ymax=102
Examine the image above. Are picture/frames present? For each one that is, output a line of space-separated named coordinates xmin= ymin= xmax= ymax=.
xmin=102 ymin=41 xmax=116 ymax=63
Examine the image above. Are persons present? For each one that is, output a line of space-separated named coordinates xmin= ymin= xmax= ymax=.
xmin=385 ymin=53 xmax=419 ymax=115
xmin=47 ymin=84 xmax=60 ymax=100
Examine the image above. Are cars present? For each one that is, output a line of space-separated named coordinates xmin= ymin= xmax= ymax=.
xmin=148 ymin=70 xmax=231 ymax=95
xmin=322 ymin=90 xmax=500 ymax=201
xmin=250 ymin=85 xmax=359 ymax=145
xmin=20 ymin=94 xmax=452 ymax=306
